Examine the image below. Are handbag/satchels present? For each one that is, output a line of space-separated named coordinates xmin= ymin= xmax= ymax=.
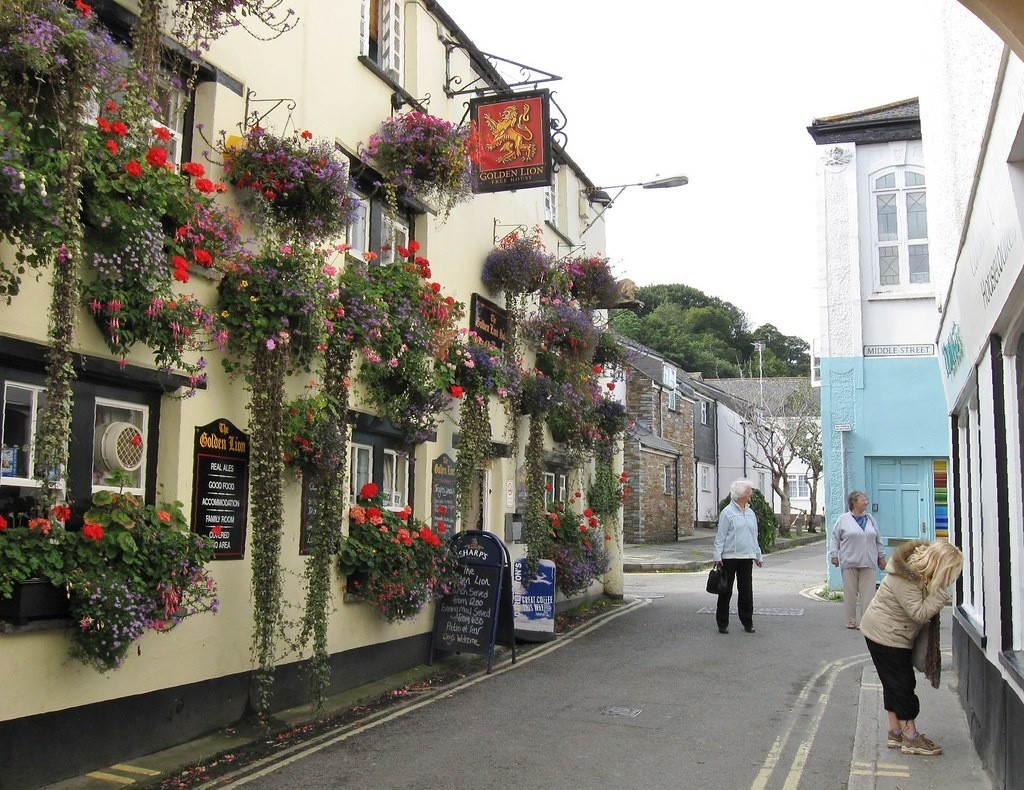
xmin=706 ymin=563 xmax=721 ymax=594
xmin=912 ymin=621 xmax=931 ymax=675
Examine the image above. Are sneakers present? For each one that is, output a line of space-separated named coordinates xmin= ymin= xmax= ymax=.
xmin=887 ymin=729 xmax=904 ymax=749
xmin=899 ymin=731 xmax=943 ymax=756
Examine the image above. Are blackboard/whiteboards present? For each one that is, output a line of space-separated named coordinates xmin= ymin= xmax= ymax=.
xmin=428 ymin=529 xmax=505 ymax=657
xmin=297 ymin=466 xmax=341 ymax=555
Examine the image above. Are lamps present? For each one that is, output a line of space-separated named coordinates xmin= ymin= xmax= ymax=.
xmin=583 ymin=190 xmax=614 ymax=203
xmin=581 ymin=155 xmax=687 ymax=190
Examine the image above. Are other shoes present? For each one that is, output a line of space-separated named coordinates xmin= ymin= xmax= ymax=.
xmin=718 ymin=627 xmax=729 ymax=634
xmin=856 ymin=624 xmax=860 ymax=630
xmin=846 ymin=622 xmax=856 ymax=629
xmin=745 ymin=627 xmax=755 ymax=633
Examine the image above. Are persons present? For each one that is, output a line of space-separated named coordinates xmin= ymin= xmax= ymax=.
xmin=711 ymin=479 xmax=764 ymax=634
xmin=830 ymin=491 xmax=886 ymax=631
xmin=858 ymin=538 xmax=965 ymax=755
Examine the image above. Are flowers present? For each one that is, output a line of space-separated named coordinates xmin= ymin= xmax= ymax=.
xmin=0 ymin=0 xmax=631 ymax=741
